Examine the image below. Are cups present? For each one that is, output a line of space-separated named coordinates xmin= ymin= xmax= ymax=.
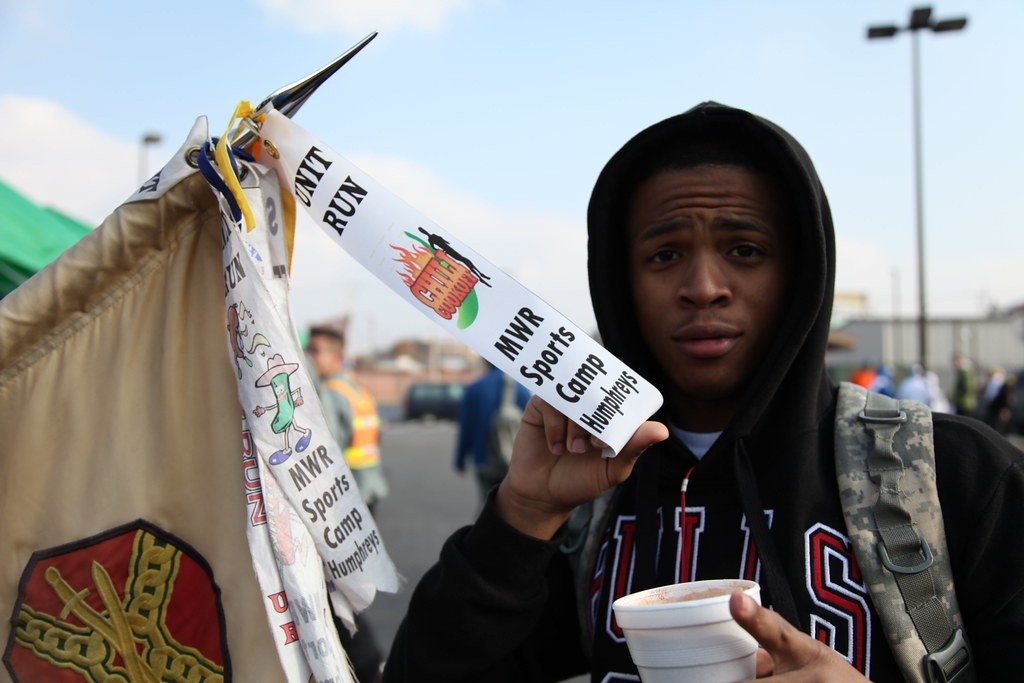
xmin=613 ymin=579 xmax=762 ymax=683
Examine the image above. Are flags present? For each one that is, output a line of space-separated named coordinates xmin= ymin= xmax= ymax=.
xmin=0 ymin=116 xmax=345 ymax=682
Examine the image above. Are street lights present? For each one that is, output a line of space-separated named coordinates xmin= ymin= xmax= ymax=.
xmin=867 ymin=7 xmax=969 ymax=377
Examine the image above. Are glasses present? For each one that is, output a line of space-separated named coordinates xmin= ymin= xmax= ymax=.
xmin=306 ymin=345 xmax=336 ymax=354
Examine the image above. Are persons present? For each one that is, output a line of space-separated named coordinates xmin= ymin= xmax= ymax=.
xmin=300 ymin=323 xmax=389 ymax=683
xmin=852 ymin=352 xmax=1024 ymax=436
xmin=452 ymin=352 xmax=539 ymax=516
xmin=376 ymin=97 xmax=1024 ymax=683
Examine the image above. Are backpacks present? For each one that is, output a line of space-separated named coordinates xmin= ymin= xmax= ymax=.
xmin=484 ymin=371 xmax=524 ymax=478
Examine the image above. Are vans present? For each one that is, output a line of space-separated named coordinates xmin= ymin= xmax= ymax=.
xmin=405 ymin=383 xmax=466 ymax=421
